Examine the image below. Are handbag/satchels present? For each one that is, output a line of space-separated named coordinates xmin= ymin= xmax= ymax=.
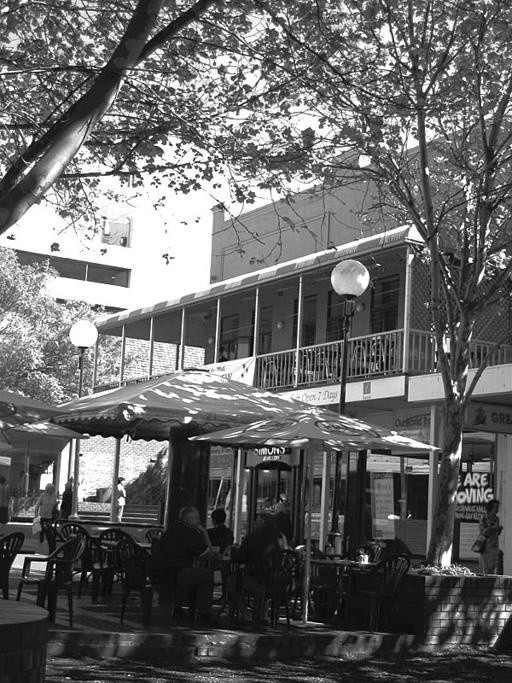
xmin=472 ymin=539 xmax=485 ymax=553
xmin=32 ymin=522 xmax=42 ymax=536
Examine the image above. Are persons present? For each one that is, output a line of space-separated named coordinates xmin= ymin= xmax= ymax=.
xmin=151 ymin=503 xmax=299 ymax=628
xmin=470 ymin=498 xmax=503 ymax=574
xmin=0 ymin=473 xmax=134 ymax=545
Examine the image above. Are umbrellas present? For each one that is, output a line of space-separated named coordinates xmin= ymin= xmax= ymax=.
xmin=187 ymin=407 xmax=436 ymax=632
xmin=40 ymin=365 xmax=319 ymax=609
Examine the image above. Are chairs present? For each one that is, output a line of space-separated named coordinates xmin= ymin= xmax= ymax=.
xmin=261 ymin=334 xmax=396 ymax=391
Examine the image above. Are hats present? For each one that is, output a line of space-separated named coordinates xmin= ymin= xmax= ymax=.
xmin=118 ymin=477 xmax=125 ymax=483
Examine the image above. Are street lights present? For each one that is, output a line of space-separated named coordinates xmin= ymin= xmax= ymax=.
xmin=329 ymin=256 xmax=370 ymax=563
xmin=68 ymin=315 xmax=100 ymax=522
